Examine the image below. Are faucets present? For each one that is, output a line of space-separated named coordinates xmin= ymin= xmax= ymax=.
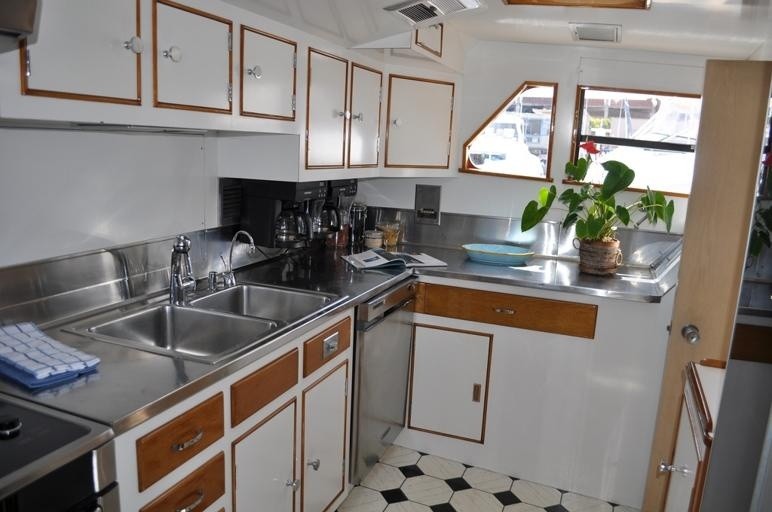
xmin=220 ymin=230 xmax=256 ymax=290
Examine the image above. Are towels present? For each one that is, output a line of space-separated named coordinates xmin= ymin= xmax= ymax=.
xmin=0 ymin=322 xmax=101 ymax=394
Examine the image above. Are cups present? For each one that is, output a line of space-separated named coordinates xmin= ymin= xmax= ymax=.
xmin=376 ymin=223 xmax=400 ymax=247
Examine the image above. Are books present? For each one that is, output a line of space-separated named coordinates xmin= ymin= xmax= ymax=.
xmin=340 ymin=247 xmax=448 ymax=270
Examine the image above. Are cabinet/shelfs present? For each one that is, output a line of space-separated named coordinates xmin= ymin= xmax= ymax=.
xmin=393 ymin=270 xmax=598 ymax=498
xmin=655 ymin=358 xmax=726 ymax=511
xmin=112 ymin=302 xmax=357 ymax=512
xmin=217 ymin=38 xmax=385 ymax=183
xmin=1 ymin=1 xmax=236 ymax=137
xmin=378 ymin=64 xmax=463 ymax=178
xmin=236 ymin=6 xmax=302 ymax=135
xmin=350 ymin=21 xmax=445 ymax=63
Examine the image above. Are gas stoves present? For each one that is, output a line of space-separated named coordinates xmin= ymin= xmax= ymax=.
xmin=1 ymin=395 xmax=112 ymax=487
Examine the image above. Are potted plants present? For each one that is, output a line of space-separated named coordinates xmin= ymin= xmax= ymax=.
xmin=520 ymin=156 xmax=673 ymax=277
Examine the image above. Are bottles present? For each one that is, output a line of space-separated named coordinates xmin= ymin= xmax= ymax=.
xmin=324 ymin=210 xmax=349 ymax=247
xmin=350 ymin=202 xmax=367 ymax=244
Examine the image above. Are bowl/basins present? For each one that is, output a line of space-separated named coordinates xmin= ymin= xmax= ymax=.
xmin=460 ymin=242 xmax=534 ymax=266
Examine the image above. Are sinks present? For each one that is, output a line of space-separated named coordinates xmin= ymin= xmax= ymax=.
xmin=59 ymin=302 xmax=287 ymax=367
xmin=185 ymin=282 xmax=349 ymax=323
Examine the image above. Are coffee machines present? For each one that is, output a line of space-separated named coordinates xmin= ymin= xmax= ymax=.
xmin=237 ymin=180 xmax=327 ymax=247
xmin=326 ymin=178 xmax=358 ymax=209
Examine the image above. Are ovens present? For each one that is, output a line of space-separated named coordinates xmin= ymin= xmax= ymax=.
xmin=1 ymin=440 xmax=122 ymax=512
